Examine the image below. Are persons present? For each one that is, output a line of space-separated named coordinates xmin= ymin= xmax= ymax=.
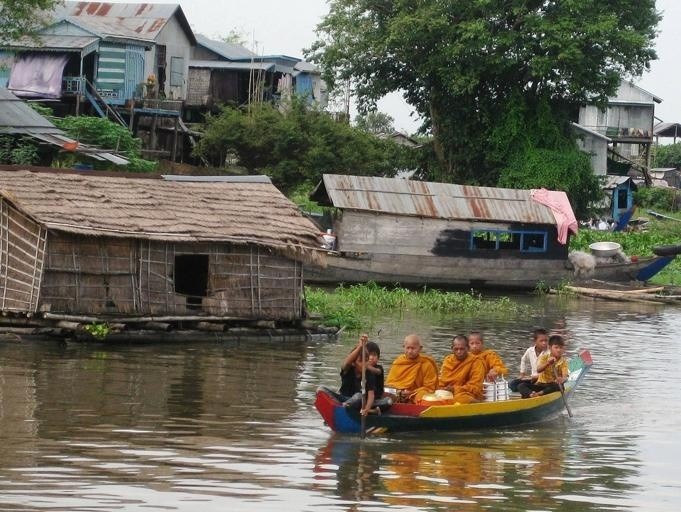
xmin=355 ymin=341 xmax=395 ymax=417
xmin=508 ymin=329 xmax=551 ymax=391
xmin=384 ymin=334 xmax=440 ymax=405
xmin=467 ymin=331 xmax=510 ymax=383
xmin=437 ymin=336 xmax=488 ymax=403
xmin=337 ymin=333 xmax=384 ymax=419
xmin=516 ymin=336 xmax=569 ymax=399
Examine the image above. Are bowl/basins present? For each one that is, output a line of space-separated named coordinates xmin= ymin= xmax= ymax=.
xmin=589 ymin=241 xmax=622 ymax=257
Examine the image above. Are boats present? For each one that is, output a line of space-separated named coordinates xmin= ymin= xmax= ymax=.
xmin=302 ymin=171 xmax=676 ymax=289
xmin=317 ymin=350 xmax=593 ymax=431
